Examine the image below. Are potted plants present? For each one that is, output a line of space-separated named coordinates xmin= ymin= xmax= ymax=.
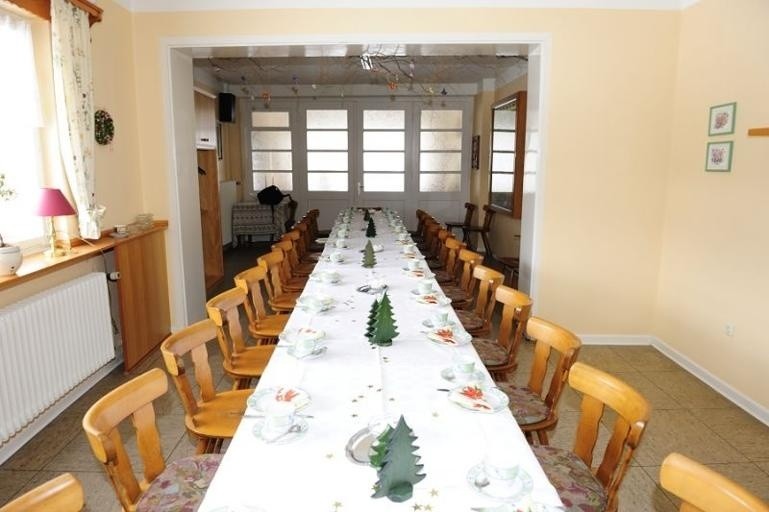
xmin=0 ymin=172 xmax=24 ymax=278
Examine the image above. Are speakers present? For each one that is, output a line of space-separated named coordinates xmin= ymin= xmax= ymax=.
xmin=218 ymin=92 xmax=236 ymax=123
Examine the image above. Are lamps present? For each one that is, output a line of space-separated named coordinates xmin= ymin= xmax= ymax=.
xmin=35 ymin=187 xmax=79 ymax=259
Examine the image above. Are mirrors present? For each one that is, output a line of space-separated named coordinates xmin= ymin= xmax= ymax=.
xmin=486 ymin=89 xmax=527 ymax=220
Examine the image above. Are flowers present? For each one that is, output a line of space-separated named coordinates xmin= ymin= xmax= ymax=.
xmin=93 ymin=109 xmax=116 ymax=145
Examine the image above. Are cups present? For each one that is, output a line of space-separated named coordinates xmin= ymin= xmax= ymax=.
xmin=488 ymin=458 xmax=521 ymax=487
xmin=0 ymin=247 xmax=23 ymax=276
xmin=113 ymin=212 xmax=155 ymax=238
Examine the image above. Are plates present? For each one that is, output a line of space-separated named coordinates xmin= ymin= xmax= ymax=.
xmin=345 ymin=426 xmax=380 ymax=465
xmin=384 ymin=206 xmax=511 ymax=412
xmin=109 ymin=232 xmax=128 ymax=239
xmin=372 ymin=245 xmax=382 ymax=252
xmin=357 ymin=283 xmax=389 ymax=295
xmin=467 ymin=462 xmax=535 ymax=501
xmin=247 ymin=208 xmax=356 ymax=445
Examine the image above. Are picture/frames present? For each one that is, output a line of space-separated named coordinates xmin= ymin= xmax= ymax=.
xmin=707 ymin=101 xmax=737 ymax=137
xmin=704 ymin=140 xmax=735 ymax=174
